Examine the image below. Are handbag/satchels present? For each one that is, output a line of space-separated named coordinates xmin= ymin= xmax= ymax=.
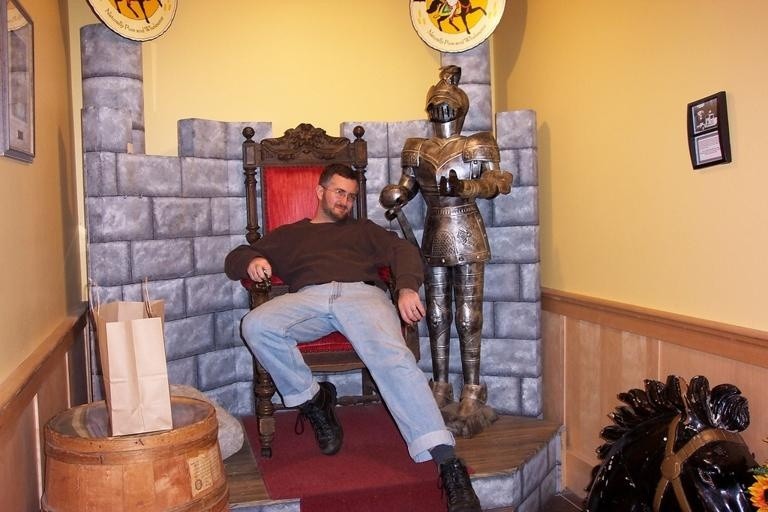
xmin=88 ymin=275 xmax=174 ymax=437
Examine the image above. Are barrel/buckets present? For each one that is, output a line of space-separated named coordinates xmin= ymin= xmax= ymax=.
xmin=40 ymin=392 xmax=231 ymax=511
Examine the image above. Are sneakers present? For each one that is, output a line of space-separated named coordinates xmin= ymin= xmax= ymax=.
xmin=296 ymin=382 xmax=344 ymax=455
xmin=437 ymin=460 xmax=481 ymax=511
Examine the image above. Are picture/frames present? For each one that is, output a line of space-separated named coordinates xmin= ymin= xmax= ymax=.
xmin=1 ymin=0 xmax=35 ymax=167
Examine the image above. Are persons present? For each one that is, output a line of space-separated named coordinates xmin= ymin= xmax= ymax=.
xmin=221 ymin=161 xmax=486 ymax=511
xmin=378 ymin=64 xmax=515 ymax=436
xmin=695 ymin=110 xmax=707 ymax=132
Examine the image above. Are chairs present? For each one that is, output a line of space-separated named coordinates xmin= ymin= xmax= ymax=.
xmin=236 ymin=119 xmax=421 ymax=457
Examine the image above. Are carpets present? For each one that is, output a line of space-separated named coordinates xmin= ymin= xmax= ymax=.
xmin=239 ymin=406 xmax=474 ymax=512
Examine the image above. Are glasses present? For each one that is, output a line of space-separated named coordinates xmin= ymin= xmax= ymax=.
xmin=322 ymin=185 xmax=358 ymax=203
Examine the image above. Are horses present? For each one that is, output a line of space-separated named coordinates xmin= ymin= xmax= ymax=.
xmin=426 ymin=0 xmax=486 ymax=35
xmin=580 ymin=374 xmax=763 ymax=511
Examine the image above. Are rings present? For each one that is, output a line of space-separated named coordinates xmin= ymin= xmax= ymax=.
xmin=255 ymin=263 xmax=262 ymax=268
